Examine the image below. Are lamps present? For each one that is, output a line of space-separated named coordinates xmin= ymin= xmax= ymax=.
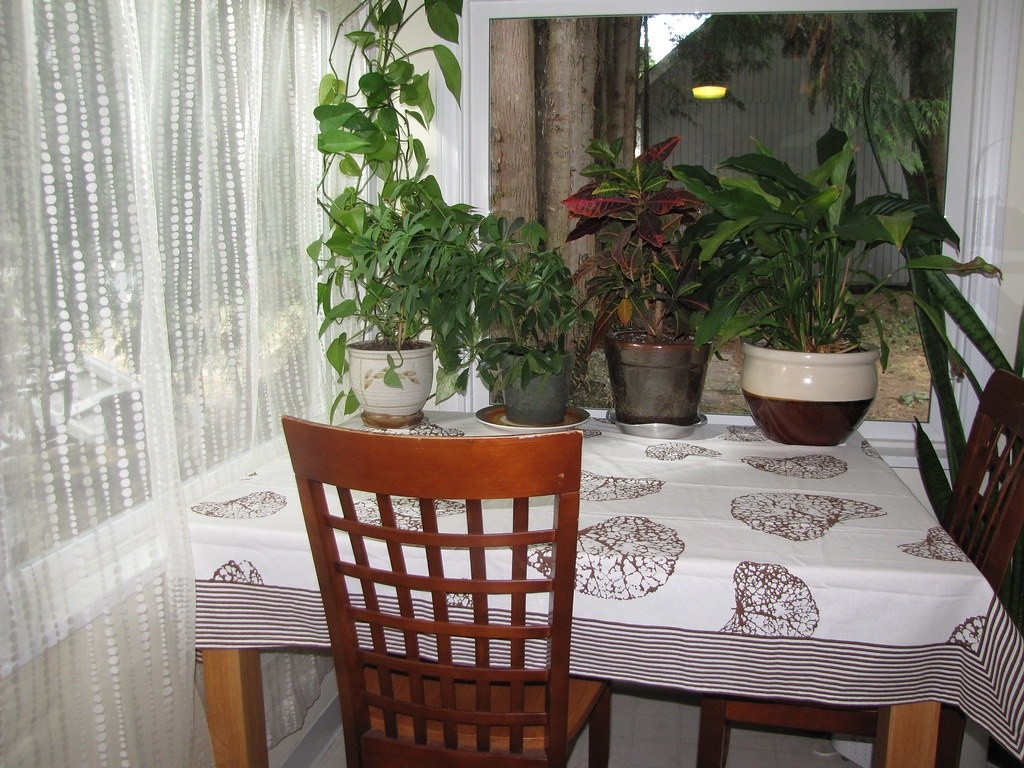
xmin=692 ymin=57 xmax=728 ymax=100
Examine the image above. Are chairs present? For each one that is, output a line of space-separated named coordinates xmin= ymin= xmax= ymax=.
xmin=281 ymin=413 xmax=611 ymax=768
xmin=696 ymin=370 xmax=1024 ymax=768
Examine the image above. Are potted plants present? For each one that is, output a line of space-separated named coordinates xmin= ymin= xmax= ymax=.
xmin=458 ymin=215 xmax=593 ymax=434
xmin=669 ymin=123 xmax=962 ymax=447
xmin=307 ymin=0 xmax=480 ymax=430
xmin=563 ymin=138 xmax=729 ymax=437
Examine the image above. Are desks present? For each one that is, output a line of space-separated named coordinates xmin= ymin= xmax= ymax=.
xmin=185 ymin=411 xmax=1024 ymax=768
